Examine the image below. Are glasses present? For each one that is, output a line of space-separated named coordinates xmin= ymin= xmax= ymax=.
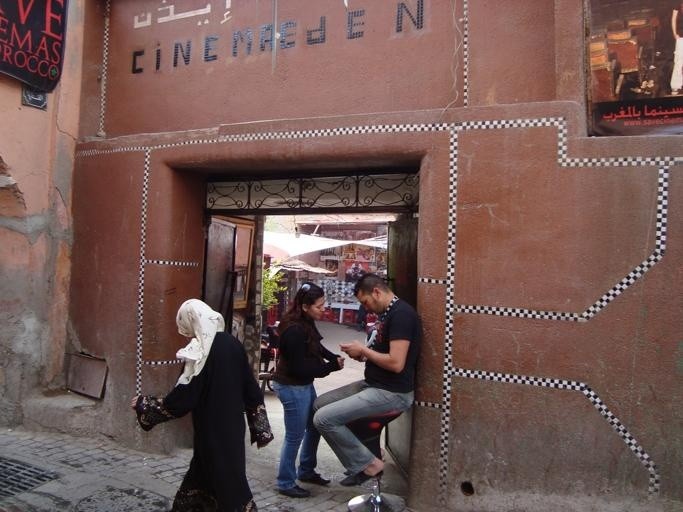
xmin=301 ymin=283 xmax=311 ymax=296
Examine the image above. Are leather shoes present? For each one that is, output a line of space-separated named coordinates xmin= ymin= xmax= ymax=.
xmin=340 ymin=470 xmax=383 ymax=487
xmin=298 ymin=471 xmax=331 ymax=486
xmin=274 ymin=485 xmax=310 ymax=497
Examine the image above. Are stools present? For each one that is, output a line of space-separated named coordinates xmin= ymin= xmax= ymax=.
xmin=348 ymin=410 xmax=407 ymax=512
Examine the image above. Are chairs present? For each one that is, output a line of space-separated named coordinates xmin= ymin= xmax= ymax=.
xmin=591 ymin=5 xmax=660 ymax=104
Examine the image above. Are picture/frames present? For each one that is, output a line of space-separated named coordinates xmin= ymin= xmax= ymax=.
xmin=583 ymin=0 xmax=683 ymax=136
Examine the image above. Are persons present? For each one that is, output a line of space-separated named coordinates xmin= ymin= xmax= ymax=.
xmin=312 ymin=269 xmax=424 ymax=488
xmin=363 ymin=312 xmax=378 ymax=342
xmin=668 ymin=1 xmax=683 ymax=98
xmin=129 ymin=297 xmax=273 ymax=512
xmin=365 ymin=319 xmax=380 ymax=351
xmin=270 ymin=279 xmax=345 ymax=499
